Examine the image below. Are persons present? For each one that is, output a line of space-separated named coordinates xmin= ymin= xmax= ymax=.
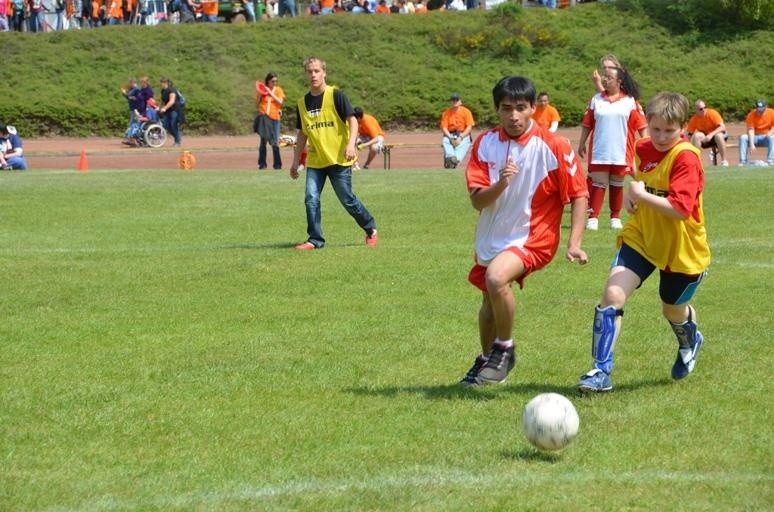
xmin=578 ymin=66 xmax=649 ymax=234
xmin=590 ymin=52 xmax=643 ymax=182
xmin=738 ymin=98 xmax=774 ymax=167
xmin=532 ymin=92 xmax=561 ymax=131
xmin=253 ymin=71 xmax=286 ymax=171
xmin=120 ymin=75 xmax=183 ymax=150
xmin=0 ymin=122 xmax=28 ymax=170
xmin=352 ymin=106 xmax=386 ymax=170
xmin=0 ymin=0 xmax=577 ymax=33
xmin=685 ymin=99 xmax=730 ymax=167
xmin=440 ymin=92 xmax=475 ymax=169
xmin=459 ymin=75 xmax=591 ymax=391
xmin=578 ymin=90 xmax=712 ymax=392
xmin=288 ymin=55 xmax=382 ymax=252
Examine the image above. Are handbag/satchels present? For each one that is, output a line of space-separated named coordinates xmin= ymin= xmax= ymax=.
xmin=254 ymin=116 xmax=260 ymax=134
xmin=175 ymin=90 xmax=186 ymax=107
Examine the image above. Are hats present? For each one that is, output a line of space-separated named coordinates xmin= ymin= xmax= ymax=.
xmin=256 ymin=80 xmax=269 ymax=97
xmin=6 ymin=123 xmax=17 ymax=135
xmin=450 ymin=93 xmax=460 ymax=99
xmin=756 ymin=99 xmax=766 ymax=113
xmin=146 ymin=98 xmax=158 ymax=108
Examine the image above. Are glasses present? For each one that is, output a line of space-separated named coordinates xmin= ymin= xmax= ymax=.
xmin=452 ymin=99 xmax=459 ymax=101
xmin=700 ymin=107 xmax=706 ymax=110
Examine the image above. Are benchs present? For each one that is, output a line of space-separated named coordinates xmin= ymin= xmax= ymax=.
xmin=712 ymin=143 xmax=739 ymax=165
xmin=380 ymin=144 xmax=394 ymax=170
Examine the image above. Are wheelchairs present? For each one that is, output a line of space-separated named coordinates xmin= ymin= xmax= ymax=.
xmin=127 ymin=118 xmax=167 ymax=148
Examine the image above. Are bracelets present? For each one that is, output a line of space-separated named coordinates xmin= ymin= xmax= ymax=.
xmin=356 ymin=144 xmax=364 ymax=152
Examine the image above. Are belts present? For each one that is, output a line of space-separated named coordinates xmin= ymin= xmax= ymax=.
xmin=449 ymin=131 xmax=464 ymax=134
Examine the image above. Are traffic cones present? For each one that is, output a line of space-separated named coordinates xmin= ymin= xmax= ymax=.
xmin=78 ymin=149 xmax=90 ymax=170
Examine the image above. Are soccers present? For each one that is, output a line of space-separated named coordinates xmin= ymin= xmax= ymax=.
xmin=522 ymin=392 xmax=580 ymax=449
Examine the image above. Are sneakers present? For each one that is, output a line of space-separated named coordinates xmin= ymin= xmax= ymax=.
xmin=273 ymin=164 xmax=281 ymax=169
xmin=721 ymin=159 xmax=728 ymax=167
xmin=295 ymin=240 xmax=324 ymax=250
xmin=258 ymin=163 xmax=267 ymax=169
xmin=445 ymin=157 xmax=457 ymax=168
xmin=366 ymin=218 xmax=377 ymax=246
xmin=672 ymin=332 xmax=703 ymax=380
xmin=579 ymin=368 xmax=614 ymax=392
xmin=610 ymin=218 xmax=623 ymax=229
xmin=458 ymin=355 xmax=489 ymax=387
xmin=738 ymin=161 xmax=746 ymax=167
xmin=586 ymin=218 xmax=598 ymax=231
xmin=477 ymin=344 xmax=515 ymax=384
xmin=767 ymin=160 xmax=774 ymax=167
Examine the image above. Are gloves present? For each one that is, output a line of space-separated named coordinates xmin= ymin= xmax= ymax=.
xmin=357 ymin=142 xmax=364 ymax=152
